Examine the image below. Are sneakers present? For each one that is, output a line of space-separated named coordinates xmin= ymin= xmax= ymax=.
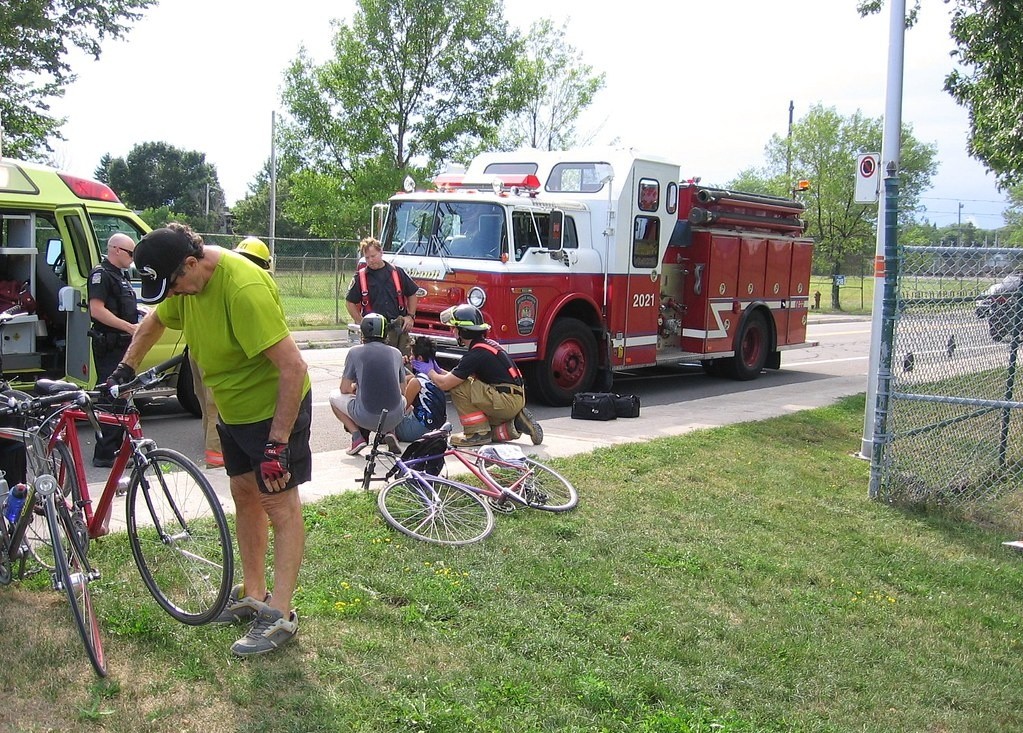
xmin=450 ymin=431 xmax=492 ymax=446
xmin=385 ymin=432 xmax=401 ymax=453
xmin=230 ymin=604 xmax=298 ymax=654
xmin=208 ymin=583 xmax=270 ymax=622
xmin=514 ymin=409 xmax=542 ymax=445
xmin=346 ymin=437 xmax=367 ymax=455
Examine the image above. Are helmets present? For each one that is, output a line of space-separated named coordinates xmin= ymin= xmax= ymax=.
xmin=440 ymin=304 xmax=490 ymax=330
xmin=231 ymin=237 xmax=273 ymax=263
xmin=359 ymin=313 xmax=388 ymax=339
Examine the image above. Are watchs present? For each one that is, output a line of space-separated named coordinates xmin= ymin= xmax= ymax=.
xmin=408 ymin=312 xmax=416 ymax=319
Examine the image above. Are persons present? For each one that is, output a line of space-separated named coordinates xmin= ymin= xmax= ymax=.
xmin=107 ymin=230 xmax=312 ymax=657
xmin=188 ymin=236 xmax=272 ymax=467
xmin=328 ymin=313 xmax=408 ymax=454
xmin=88 ymin=232 xmax=151 ymax=468
xmin=390 ymin=337 xmax=447 ymax=441
xmin=415 ymin=302 xmax=545 ymax=446
xmin=346 ymin=237 xmax=419 ymax=357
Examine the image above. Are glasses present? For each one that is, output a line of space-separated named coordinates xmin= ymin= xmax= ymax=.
xmin=169 ymin=263 xmax=186 ymax=288
xmin=112 ymin=246 xmax=133 ymax=257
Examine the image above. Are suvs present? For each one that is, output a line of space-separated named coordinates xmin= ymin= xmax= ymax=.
xmin=979 ymin=269 xmax=1023 ymax=341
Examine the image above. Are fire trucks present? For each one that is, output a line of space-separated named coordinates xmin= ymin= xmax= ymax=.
xmin=373 ymin=151 xmax=820 ymax=405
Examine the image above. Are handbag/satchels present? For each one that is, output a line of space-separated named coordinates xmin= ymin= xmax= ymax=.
xmin=571 ymin=392 xmax=616 ymax=420
xmin=607 ymin=392 xmax=639 ymax=417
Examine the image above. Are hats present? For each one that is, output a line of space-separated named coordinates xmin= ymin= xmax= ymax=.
xmin=132 ymin=229 xmax=190 ymax=304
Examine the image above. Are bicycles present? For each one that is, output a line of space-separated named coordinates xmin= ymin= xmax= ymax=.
xmin=16 ymin=354 xmax=233 ymax=626
xmin=0 ymin=388 xmax=108 ymax=678
xmin=362 ymin=408 xmax=579 ymax=544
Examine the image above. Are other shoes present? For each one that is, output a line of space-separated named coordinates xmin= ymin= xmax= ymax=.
xmin=93 ymin=456 xmax=134 ymax=469
xmin=205 ymin=452 xmax=224 ymax=470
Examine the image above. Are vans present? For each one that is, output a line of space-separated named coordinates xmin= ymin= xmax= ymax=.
xmin=1 ymin=157 xmax=203 ymax=422
